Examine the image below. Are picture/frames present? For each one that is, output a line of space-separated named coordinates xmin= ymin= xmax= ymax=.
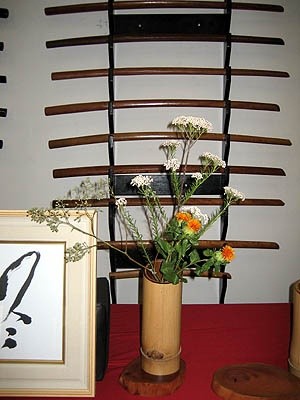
xmin=0 ymin=210 xmax=99 ymax=397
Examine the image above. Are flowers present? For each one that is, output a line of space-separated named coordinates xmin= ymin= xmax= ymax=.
xmin=26 ymin=116 xmax=246 ymax=281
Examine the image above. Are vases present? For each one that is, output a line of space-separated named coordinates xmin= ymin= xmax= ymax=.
xmin=140 ymin=259 xmax=184 ymax=377
